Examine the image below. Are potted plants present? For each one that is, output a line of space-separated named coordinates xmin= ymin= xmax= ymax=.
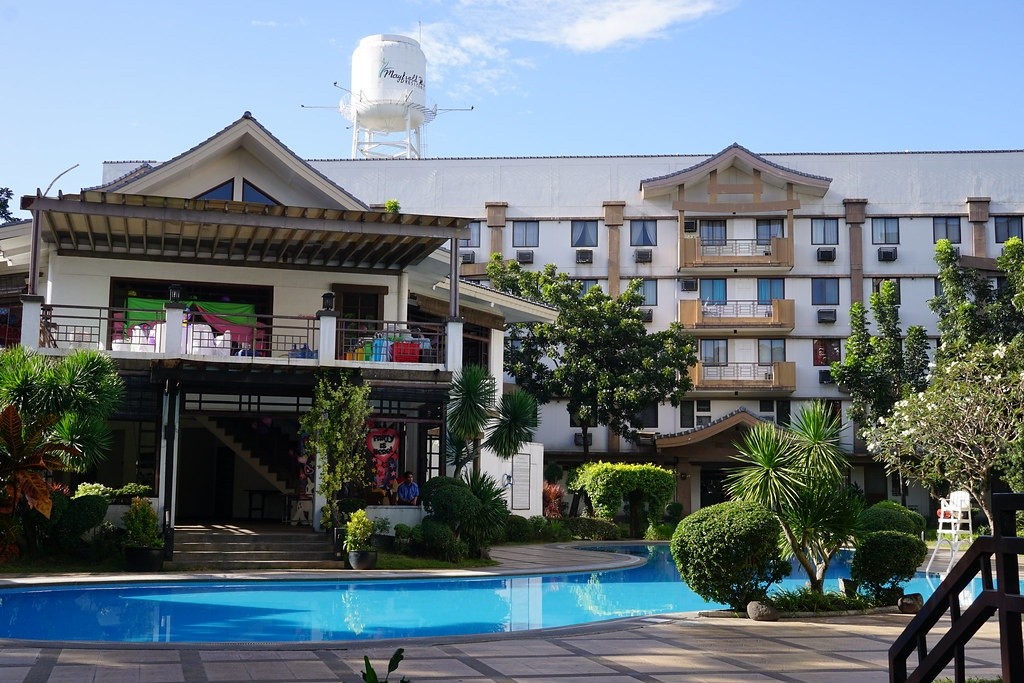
xmin=339 ymin=505 xmax=378 ymax=569
xmin=119 ymin=494 xmax=167 ymax=575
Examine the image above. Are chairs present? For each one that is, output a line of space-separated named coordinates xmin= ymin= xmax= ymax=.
xmin=937 ymin=490 xmax=972 ymax=551
xmin=130 ymin=322 xmax=229 ymax=355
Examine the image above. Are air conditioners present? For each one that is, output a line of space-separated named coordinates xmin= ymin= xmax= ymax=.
xmin=460 ymin=220 xmax=897 ymax=448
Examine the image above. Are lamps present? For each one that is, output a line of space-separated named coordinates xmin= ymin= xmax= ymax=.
xmin=40 ymin=305 xmax=52 ymax=321
xmin=169 ymin=283 xmax=181 ymax=302
xmin=321 ymin=292 xmax=336 ymax=310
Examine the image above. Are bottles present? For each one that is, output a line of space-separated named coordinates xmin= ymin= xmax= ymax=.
xmin=301 ymin=343 xmax=312 ymax=358
xmin=288 ymin=344 xmax=301 ymax=358
xmin=313 ymin=349 xmax=318 ymax=358
xmin=404 ymin=337 xmax=431 ymax=348
xmin=347 ymin=333 xmax=390 ymax=362
xmin=235 ymin=342 xmax=260 ymax=357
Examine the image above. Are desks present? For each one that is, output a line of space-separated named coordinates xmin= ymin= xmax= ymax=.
xmin=153 ymin=322 xmax=191 ymax=355
xmin=240 ymin=488 xmax=281 ymax=525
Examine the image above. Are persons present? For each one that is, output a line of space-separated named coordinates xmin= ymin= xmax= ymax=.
xmin=397 ymin=471 xmax=419 ymax=506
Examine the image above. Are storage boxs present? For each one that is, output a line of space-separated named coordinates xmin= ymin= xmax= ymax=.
xmin=389 ymin=342 xmax=420 ymax=362
